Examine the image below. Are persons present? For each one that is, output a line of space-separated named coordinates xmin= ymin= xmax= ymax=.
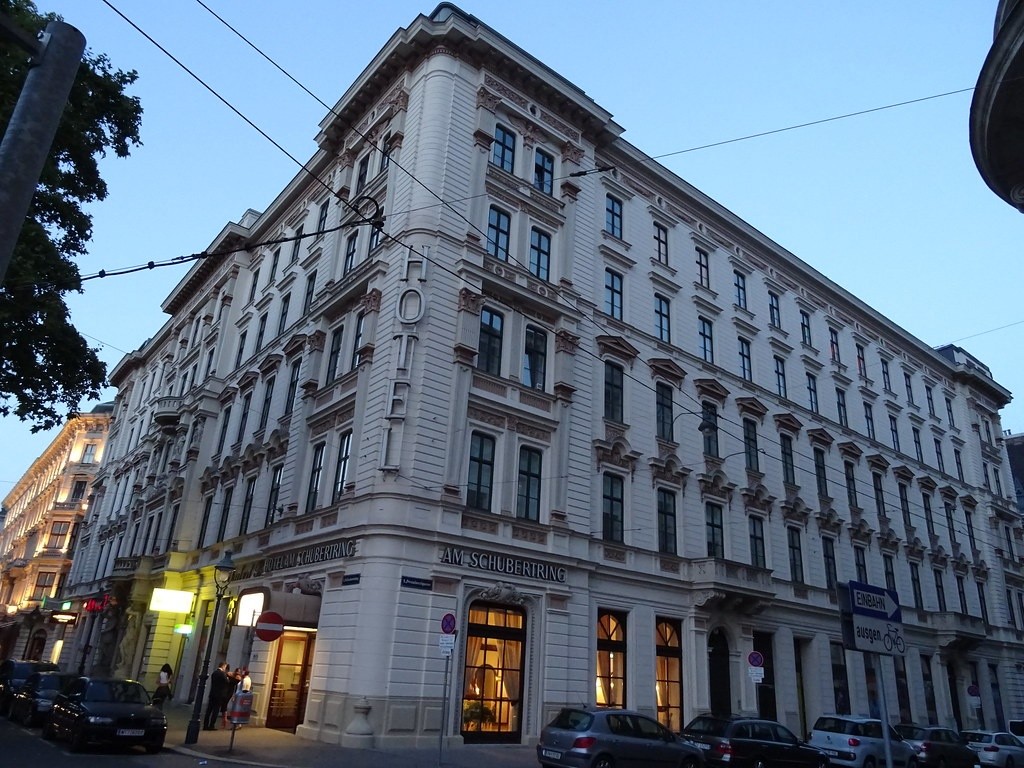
xmin=153 ymin=664 xmax=172 ymax=709
xmin=203 ymin=662 xmax=229 ymax=730
xmin=220 ymin=665 xmax=251 ymax=730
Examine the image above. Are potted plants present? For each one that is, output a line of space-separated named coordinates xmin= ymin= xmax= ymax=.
xmin=463 ymin=702 xmax=496 ymax=731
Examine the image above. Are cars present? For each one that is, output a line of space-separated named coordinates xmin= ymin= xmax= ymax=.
xmin=8 ymin=672 xmax=78 ymax=730
xmin=536 ymin=707 xmax=704 ymax=768
xmin=45 ymin=676 xmax=168 ymax=754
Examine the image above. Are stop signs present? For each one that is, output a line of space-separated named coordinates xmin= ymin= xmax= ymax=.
xmin=256 ymin=611 xmax=285 ymax=642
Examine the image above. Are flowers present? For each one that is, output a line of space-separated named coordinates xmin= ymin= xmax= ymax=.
xmin=463 ymin=699 xmax=476 ymax=711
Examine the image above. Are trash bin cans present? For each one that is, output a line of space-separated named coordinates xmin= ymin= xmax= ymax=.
xmin=230 ymin=690 xmax=253 ymax=725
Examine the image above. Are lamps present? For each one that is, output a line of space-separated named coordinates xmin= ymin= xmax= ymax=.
xmin=148 ymin=588 xmax=200 ymax=617
xmin=173 ymin=623 xmax=193 ymax=642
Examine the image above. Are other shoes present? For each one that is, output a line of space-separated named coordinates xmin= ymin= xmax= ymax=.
xmin=208 ymin=728 xmax=218 ymax=731
xmin=203 ymin=727 xmax=208 ymax=730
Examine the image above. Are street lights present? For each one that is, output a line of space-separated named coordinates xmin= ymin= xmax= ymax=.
xmin=185 ymin=550 xmax=237 ymax=744
xmin=78 ymin=586 xmax=106 ymax=674
xmin=21 ymin=605 xmax=42 ymax=660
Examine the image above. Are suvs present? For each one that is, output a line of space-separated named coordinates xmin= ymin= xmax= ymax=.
xmin=0 ymin=660 xmax=62 ymax=716
xmin=677 ymin=712 xmax=830 ymax=768
xmin=894 ymin=722 xmax=980 ymax=768
xmin=960 ymin=730 xmax=1024 ymax=768
xmin=808 ymin=714 xmax=919 ymax=768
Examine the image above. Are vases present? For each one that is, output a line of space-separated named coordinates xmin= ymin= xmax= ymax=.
xmin=465 ymin=722 xmax=470 ymax=731
